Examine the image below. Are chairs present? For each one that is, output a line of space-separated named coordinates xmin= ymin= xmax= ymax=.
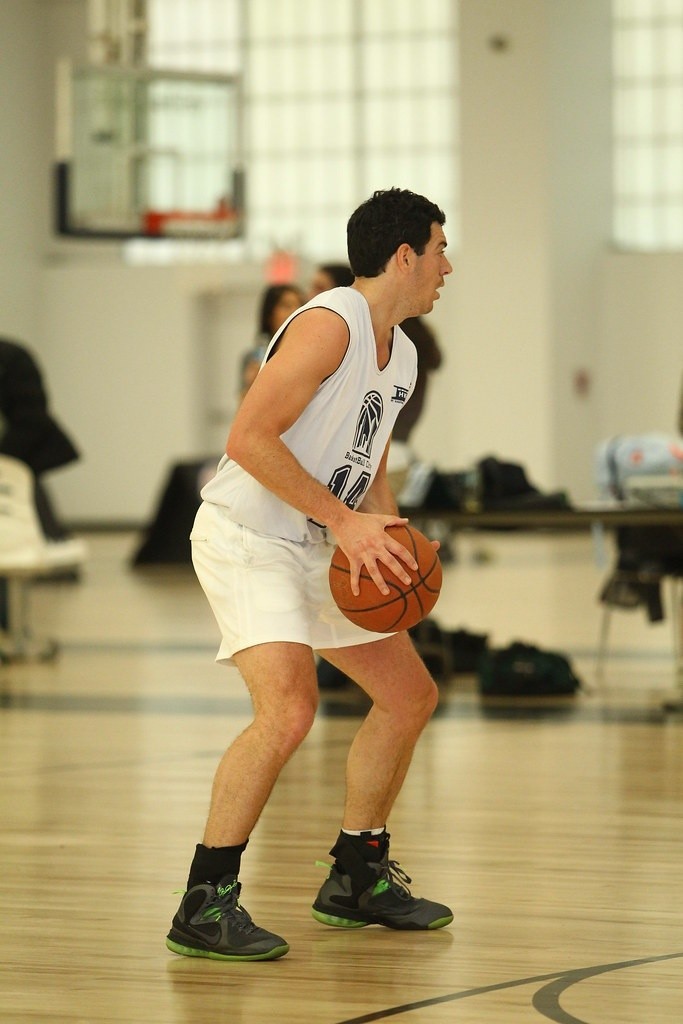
xmin=0 ymin=457 xmax=88 ymax=663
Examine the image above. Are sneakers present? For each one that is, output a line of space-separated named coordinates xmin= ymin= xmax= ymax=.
xmin=165 ymin=878 xmax=288 ymax=960
xmin=311 ymin=845 xmax=455 ymax=930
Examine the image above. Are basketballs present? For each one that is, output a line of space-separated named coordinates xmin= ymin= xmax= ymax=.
xmin=328 ymin=524 xmax=443 ymax=634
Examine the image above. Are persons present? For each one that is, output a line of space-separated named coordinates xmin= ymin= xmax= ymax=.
xmin=1 ymin=333 xmax=91 ymax=588
xmin=234 ymin=258 xmax=445 ymax=501
xmin=164 ymin=188 xmax=456 ymax=964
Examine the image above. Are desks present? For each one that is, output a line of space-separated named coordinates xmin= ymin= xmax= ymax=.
xmin=369 ymin=500 xmax=680 ymax=710
